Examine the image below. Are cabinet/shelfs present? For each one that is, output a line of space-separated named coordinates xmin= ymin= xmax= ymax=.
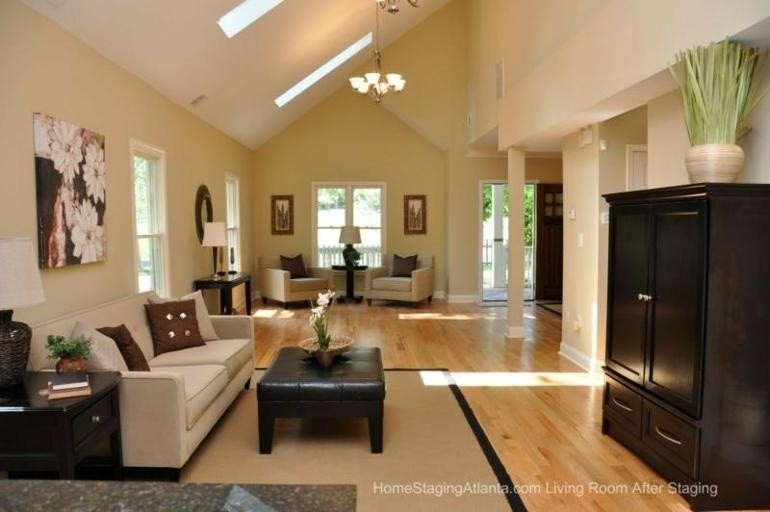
xmin=596 ymin=181 xmax=769 ymax=509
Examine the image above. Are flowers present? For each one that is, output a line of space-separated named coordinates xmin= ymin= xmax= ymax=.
xmin=34 ymin=113 xmax=108 ymax=267
xmin=304 ymin=288 xmax=338 ymax=356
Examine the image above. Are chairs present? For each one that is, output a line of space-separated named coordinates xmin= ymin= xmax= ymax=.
xmin=365 ymin=246 xmax=437 ymax=308
xmin=254 ymin=244 xmax=336 ymax=311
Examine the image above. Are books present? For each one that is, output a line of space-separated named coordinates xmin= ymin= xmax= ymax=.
xmin=48 ymin=381 xmax=91 ymax=400
xmin=53 ymin=372 xmax=88 ymax=390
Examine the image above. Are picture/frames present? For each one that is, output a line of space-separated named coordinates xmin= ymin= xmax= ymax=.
xmin=270 ymin=194 xmax=294 ymax=236
xmin=402 ymin=192 xmax=427 ymax=237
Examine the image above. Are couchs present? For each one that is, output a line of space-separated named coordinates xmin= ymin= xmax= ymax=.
xmin=21 ymin=285 xmax=258 ymax=483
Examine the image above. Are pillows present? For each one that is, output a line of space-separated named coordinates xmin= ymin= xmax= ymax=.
xmin=277 ymin=252 xmax=308 ymax=279
xmin=390 ymin=253 xmax=419 ymax=278
xmin=141 ymin=298 xmax=207 ymax=359
xmin=145 ymin=289 xmax=223 ymax=342
xmin=96 ymin=319 xmax=152 ymax=371
xmin=66 ymin=321 xmax=133 ymax=371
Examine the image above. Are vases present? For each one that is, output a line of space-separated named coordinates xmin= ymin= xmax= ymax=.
xmin=313 ymin=349 xmax=337 ymax=369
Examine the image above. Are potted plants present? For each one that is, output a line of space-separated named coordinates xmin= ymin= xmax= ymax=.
xmin=659 ymin=31 xmax=770 ymax=184
xmin=44 ymin=330 xmax=94 ymax=373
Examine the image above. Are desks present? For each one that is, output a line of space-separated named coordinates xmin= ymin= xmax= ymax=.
xmin=0 ymin=369 xmax=128 ymax=482
xmin=193 ymin=270 xmax=252 ymax=316
xmin=330 ymin=265 xmax=369 ymax=304
xmin=0 ymin=475 xmax=360 ymax=510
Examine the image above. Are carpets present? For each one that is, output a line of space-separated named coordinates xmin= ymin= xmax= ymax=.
xmin=129 ymin=370 xmax=533 ymax=511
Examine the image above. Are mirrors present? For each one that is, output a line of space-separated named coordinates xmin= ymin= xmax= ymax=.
xmin=195 ymin=183 xmax=213 ymax=246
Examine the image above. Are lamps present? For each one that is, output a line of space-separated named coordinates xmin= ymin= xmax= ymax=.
xmin=377 ymin=0 xmax=419 ymax=16
xmin=338 ymin=225 xmax=363 ymax=266
xmin=345 ymin=1 xmax=408 ymax=105
xmin=0 ymin=235 xmax=46 ymax=388
xmin=199 ymin=220 xmax=230 ymax=279
